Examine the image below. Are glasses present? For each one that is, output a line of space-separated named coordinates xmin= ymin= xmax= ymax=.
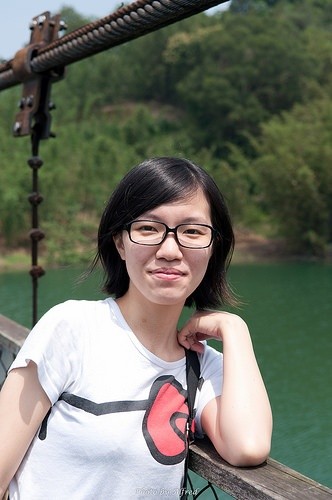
xmin=124 ymin=219 xmax=221 ymax=250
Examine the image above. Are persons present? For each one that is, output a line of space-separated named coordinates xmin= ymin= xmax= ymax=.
xmin=0 ymin=156 xmax=272 ymax=500
